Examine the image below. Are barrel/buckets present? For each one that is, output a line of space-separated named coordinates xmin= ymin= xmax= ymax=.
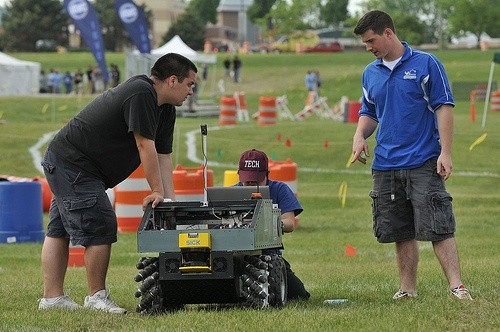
xmin=258 ymin=96 xmax=277 ymax=124
xmin=218 ymin=94 xmax=237 ymax=125
xmin=115 ymin=163 xmax=153 ymax=232
xmin=266 ymin=158 xmax=298 ymax=200
xmin=0 ymin=175 xmax=115 ymax=267
xmin=172 ymin=163 xmax=213 ymax=201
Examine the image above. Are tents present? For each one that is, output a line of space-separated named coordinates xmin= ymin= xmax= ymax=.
xmin=0 ymin=51 xmax=40 ymax=96
xmin=130 ymin=33 xmax=218 ymax=100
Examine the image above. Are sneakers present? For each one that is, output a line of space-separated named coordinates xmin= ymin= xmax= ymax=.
xmin=37 ymin=294 xmax=82 ymax=310
xmin=83 ymin=289 xmax=127 ymax=314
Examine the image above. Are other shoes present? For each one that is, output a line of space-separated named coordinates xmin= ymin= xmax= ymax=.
xmin=449 ymin=284 xmax=473 ymax=300
xmin=392 ymin=289 xmax=418 ymax=300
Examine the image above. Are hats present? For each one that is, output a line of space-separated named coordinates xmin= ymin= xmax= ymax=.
xmin=237 ymin=149 xmax=269 ymax=182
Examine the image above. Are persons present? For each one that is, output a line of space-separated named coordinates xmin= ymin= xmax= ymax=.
xmin=232 ymin=53 xmax=242 ymax=84
xmin=304 ymin=70 xmax=316 ymax=94
xmin=222 ymin=148 xmax=313 ymax=301
xmin=224 ymin=54 xmax=230 ymax=77
xmin=38 ymin=63 xmax=119 ymax=97
xmin=39 ymin=52 xmax=198 ymax=315
xmin=313 ymin=71 xmax=321 ymax=96
xmin=349 ymin=9 xmax=478 ymax=303
